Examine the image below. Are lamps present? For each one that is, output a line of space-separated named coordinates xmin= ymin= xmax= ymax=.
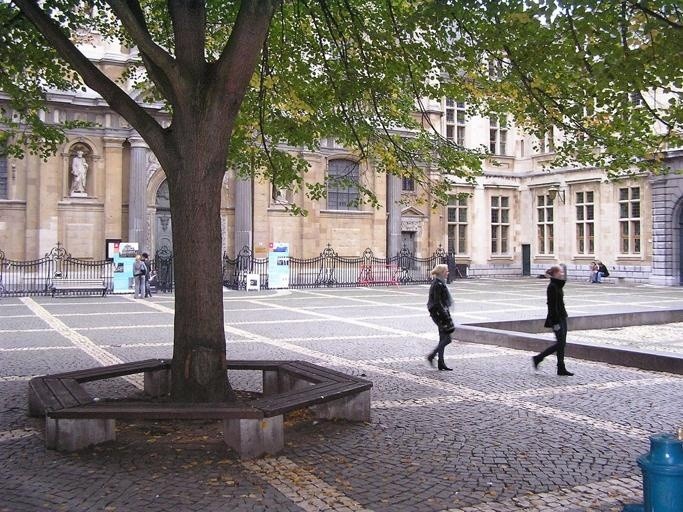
xmin=548 ymin=185 xmax=565 ymax=205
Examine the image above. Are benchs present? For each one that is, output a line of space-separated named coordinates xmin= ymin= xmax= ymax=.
xmin=30 ymin=373 xmax=119 ymax=453
xmin=154 ymin=360 xmax=281 ymax=396
xmin=590 ymin=275 xmax=625 ymax=286
xmin=49 ymin=279 xmax=107 ymax=297
xmin=278 ymin=359 xmax=373 ymax=423
xmin=29 ymin=360 xmax=165 ymax=395
xmin=254 ymin=381 xmax=373 ymax=457
xmin=47 ymin=393 xmax=267 ymax=458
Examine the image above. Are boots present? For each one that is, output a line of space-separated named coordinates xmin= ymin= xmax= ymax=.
xmin=437 ymin=358 xmax=454 ymax=371
xmin=556 ymin=361 xmax=574 ymax=375
xmin=532 ymin=353 xmax=544 ymax=368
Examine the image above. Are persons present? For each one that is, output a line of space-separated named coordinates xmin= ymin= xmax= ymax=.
xmin=587 ymin=261 xmax=597 ymax=283
xmin=141 ymin=252 xmax=152 ymax=298
xmin=70 ymin=149 xmax=90 ymax=195
xmin=531 ymin=263 xmax=577 ymax=377
xmin=426 ymin=262 xmax=456 ymax=373
xmin=132 ymin=254 xmax=147 ymax=300
xmin=592 ymin=261 xmax=608 ymax=283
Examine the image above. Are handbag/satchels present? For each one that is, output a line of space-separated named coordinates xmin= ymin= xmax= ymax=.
xmin=140 ymin=264 xmax=146 ymax=272
